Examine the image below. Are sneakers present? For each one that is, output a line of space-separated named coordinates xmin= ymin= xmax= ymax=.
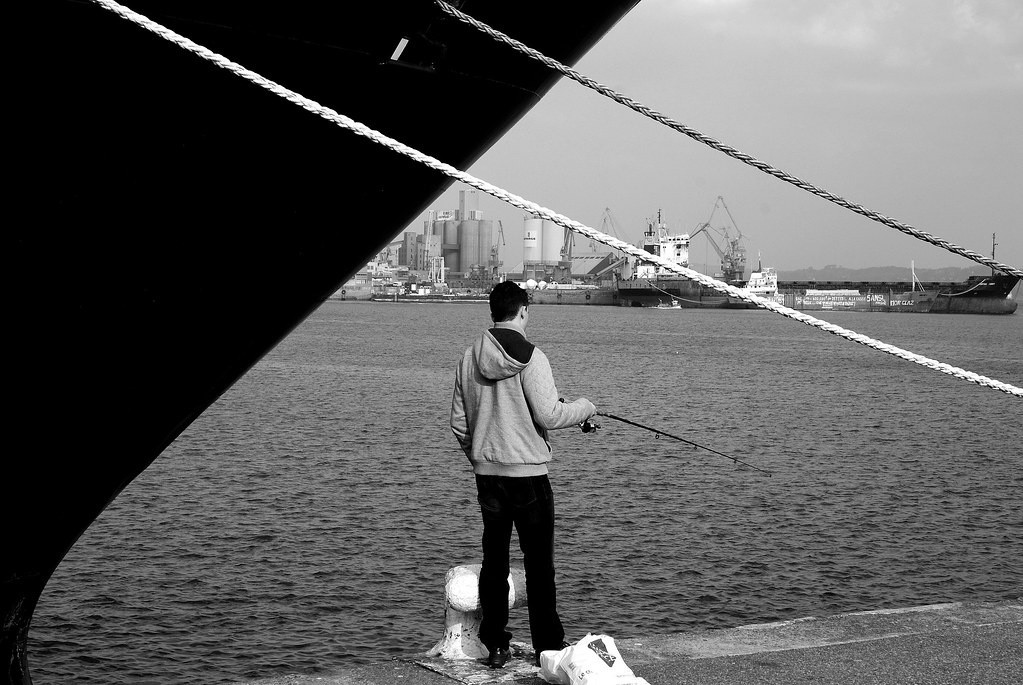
xmin=536 ymin=641 xmax=570 ymax=667
xmin=487 ymin=648 xmax=511 ymax=668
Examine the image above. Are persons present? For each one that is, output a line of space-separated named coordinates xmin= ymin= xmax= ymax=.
xmin=450 ymin=280 xmax=598 ymax=667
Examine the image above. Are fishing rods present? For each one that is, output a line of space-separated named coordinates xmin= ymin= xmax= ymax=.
xmin=557 ymin=397 xmax=772 ymax=478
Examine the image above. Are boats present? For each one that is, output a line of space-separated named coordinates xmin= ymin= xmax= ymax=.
xmin=654 ymin=295 xmax=682 ymax=310
xmin=680 ymin=196 xmax=1019 ymax=314
xmin=616 ymin=207 xmax=702 ymax=307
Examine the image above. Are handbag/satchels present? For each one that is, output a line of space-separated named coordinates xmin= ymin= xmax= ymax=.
xmin=536 ymin=632 xmax=650 ymax=685
